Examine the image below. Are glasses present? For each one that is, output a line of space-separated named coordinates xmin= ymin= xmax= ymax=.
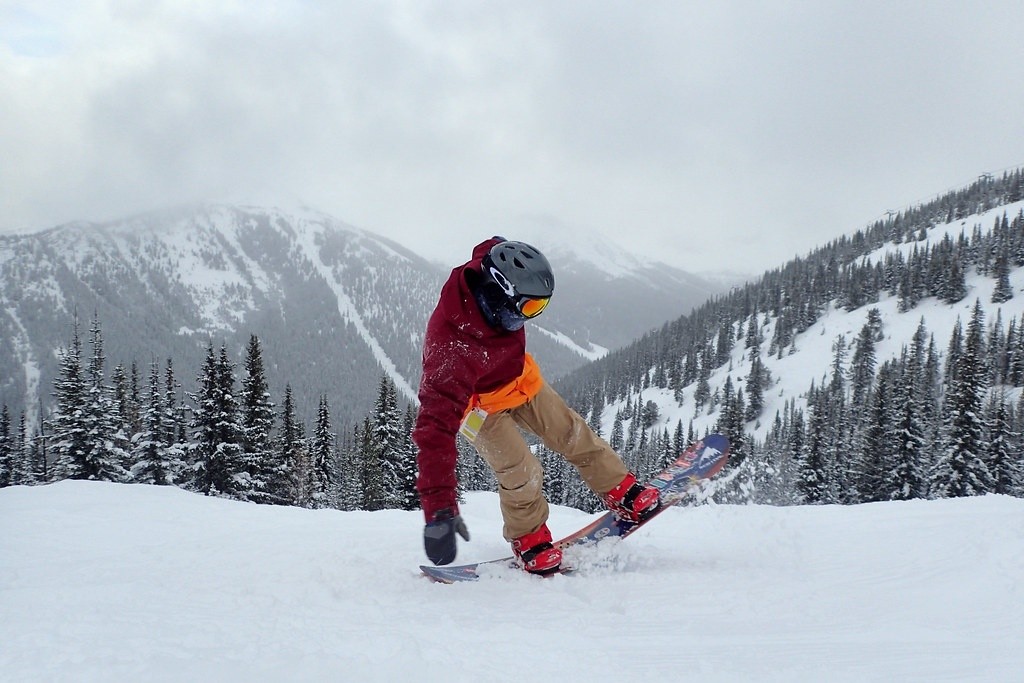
xmin=516 ymin=296 xmax=550 ymax=318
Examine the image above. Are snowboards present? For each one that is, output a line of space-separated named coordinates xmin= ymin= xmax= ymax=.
xmin=420 ymin=431 xmax=733 ymax=584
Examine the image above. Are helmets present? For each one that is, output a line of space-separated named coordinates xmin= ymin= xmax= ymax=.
xmin=480 ymin=241 xmax=555 ymax=320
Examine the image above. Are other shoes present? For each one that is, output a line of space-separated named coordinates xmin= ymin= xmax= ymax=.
xmin=608 ymin=482 xmax=658 ymax=512
xmin=515 ymin=542 xmax=562 ymax=571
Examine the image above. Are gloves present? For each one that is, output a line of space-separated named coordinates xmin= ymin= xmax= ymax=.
xmin=424 ymin=501 xmax=469 ymax=566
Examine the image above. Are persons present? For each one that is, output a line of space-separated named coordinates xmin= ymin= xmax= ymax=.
xmin=412 ymin=236 xmax=661 ymax=574
